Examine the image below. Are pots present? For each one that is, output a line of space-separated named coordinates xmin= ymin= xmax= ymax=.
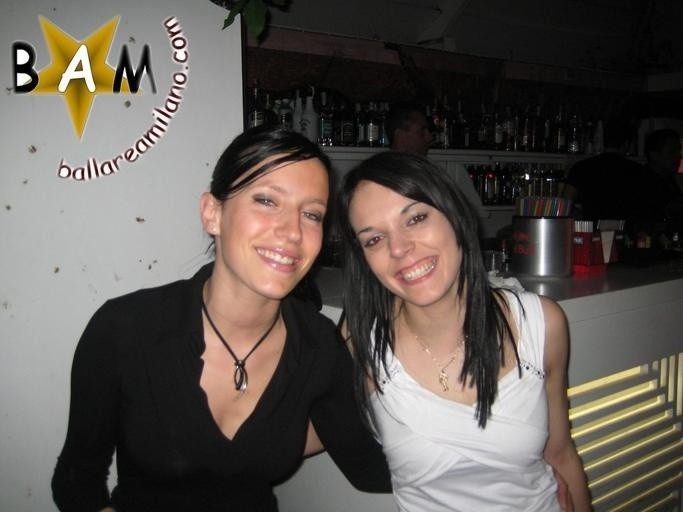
xmin=508 ymin=214 xmax=574 ymax=280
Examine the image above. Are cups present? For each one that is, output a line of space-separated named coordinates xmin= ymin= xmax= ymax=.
xmin=483 ymin=250 xmax=502 ymax=277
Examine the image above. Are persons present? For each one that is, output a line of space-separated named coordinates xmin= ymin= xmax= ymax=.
xmin=47 ymin=121 xmax=393 ymax=511
xmin=300 ymin=148 xmax=596 ymax=511
xmin=639 ymin=128 xmax=682 ymax=224
xmin=560 ymin=120 xmax=660 ymax=239
xmin=379 ymin=99 xmax=434 ymax=158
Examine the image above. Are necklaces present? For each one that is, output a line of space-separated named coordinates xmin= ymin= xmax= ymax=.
xmin=390 ymin=292 xmax=467 ymax=392
xmin=196 ymin=287 xmax=281 ymax=397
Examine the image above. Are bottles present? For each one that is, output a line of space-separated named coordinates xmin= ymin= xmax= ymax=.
xmin=494 ymin=239 xmax=513 ymax=279
xmin=461 ymin=165 xmax=565 ymax=207
xmin=247 ymin=78 xmax=596 ymax=156
xmin=624 ymin=231 xmax=652 ymax=248
